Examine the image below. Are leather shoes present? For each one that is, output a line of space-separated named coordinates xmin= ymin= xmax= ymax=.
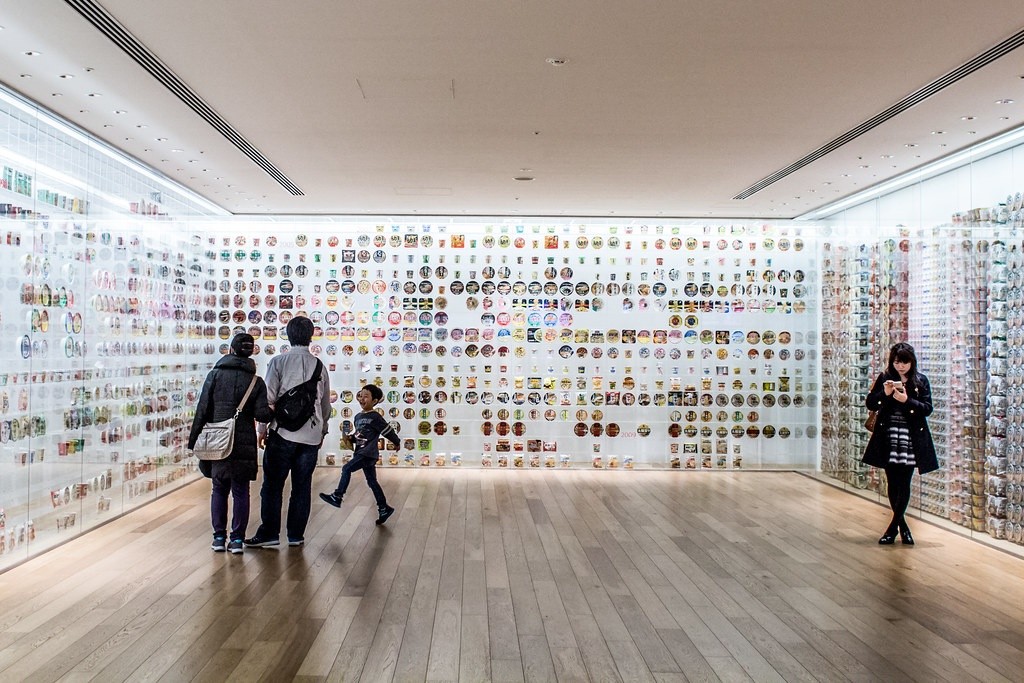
xmin=878 ymin=534 xmax=894 ymax=545
xmin=901 ymin=530 xmax=914 ymax=545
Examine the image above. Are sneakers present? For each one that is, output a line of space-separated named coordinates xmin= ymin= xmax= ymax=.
xmin=288 ymin=537 xmax=304 ymax=545
xmin=227 ymin=541 xmax=244 ymax=553
xmin=244 ymin=536 xmax=280 ymax=547
xmin=375 ymin=506 xmax=394 ymax=524
xmin=319 ymin=492 xmax=343 ymax=508
xmin=211 ymin=538 xmax=225 ymax=551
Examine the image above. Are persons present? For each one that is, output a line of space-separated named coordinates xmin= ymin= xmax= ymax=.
xmin=861 ymin=342 xmax=940 ymax=545
xmin=188 ymin=333 xmax=276 ymax=556
xmin=319 ymin=384 xmax=401 ymax=525
xmin=244 ymin=316 xmax=332 ymax=548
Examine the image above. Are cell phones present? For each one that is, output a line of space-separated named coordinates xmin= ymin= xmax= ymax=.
xmin=893 ymin=382 xmax=904 ymax=392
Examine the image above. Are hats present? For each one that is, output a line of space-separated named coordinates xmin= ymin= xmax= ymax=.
xmin=231 ymin=334 xmax=254 ymax=348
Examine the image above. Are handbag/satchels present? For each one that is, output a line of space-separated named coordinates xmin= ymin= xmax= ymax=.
xmin=193 ymin=418 xmax=235 ymax=460
xmin=864 ymin=411 xmax=878 ymax=432
xmin=274 ymin=379 xmax=317 ymax=432
